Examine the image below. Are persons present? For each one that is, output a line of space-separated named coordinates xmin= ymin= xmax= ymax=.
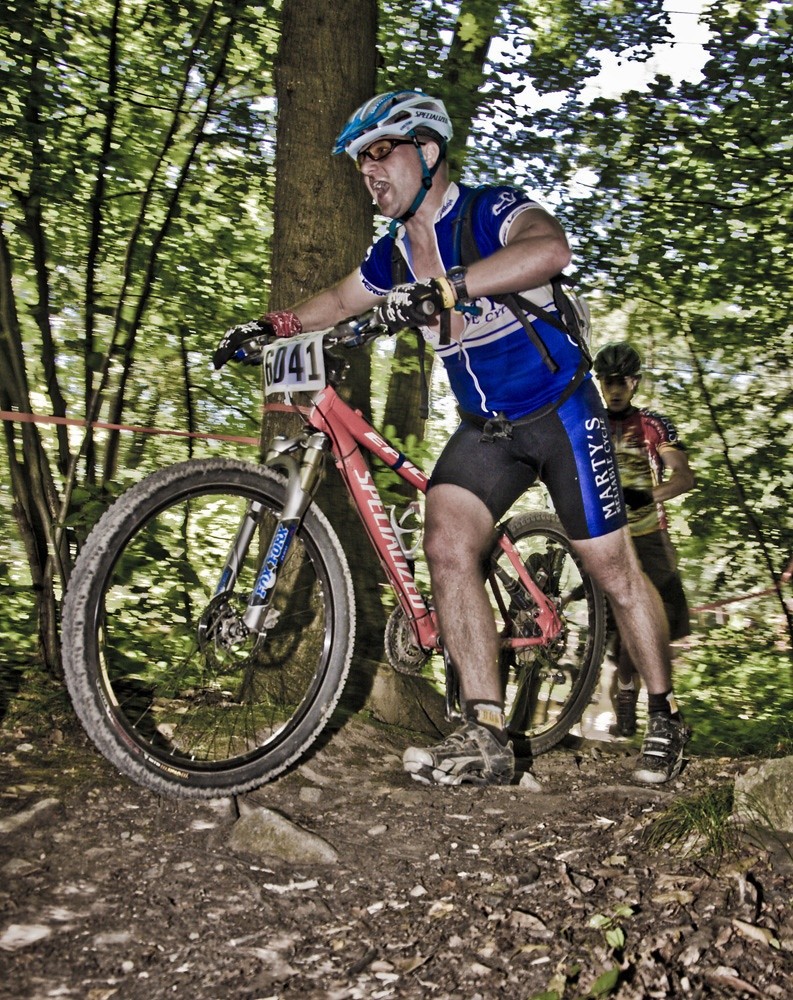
xmin=592 ymin=341 xmax=695 ymax=738
xmin=211 ymin=91 xmax=691 ymax=786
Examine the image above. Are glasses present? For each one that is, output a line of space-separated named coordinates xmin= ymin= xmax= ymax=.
xmin=353 ymin=138 xmax=429 ymax=173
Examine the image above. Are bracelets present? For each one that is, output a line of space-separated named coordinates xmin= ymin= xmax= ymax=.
xmin=262 ymin=310 xmax=303 ymax=338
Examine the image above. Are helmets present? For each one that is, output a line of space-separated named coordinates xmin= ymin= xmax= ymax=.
xmin=592 ymin=345 xmax=643 ymax=380
xmin=329 ymin=90 xmax=455 ymax=160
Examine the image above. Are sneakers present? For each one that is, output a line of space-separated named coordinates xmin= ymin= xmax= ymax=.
xmin=401 ymin=717 xmax=515 ymax=788
xmin=633 ymin=710 xmax=691 ymax=785
xmin=616 ymin=690 xmax=638 ymax=737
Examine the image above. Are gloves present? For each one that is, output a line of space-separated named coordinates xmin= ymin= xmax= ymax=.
xmin=376 ymin=274 xmax=455 ymax=337
xmin=216 ymin=317 xmax=277 ymax=367
xmin=621 ymin=483 xmax=653 ymax=513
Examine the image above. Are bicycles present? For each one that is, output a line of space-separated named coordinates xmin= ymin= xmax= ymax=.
xmin=55 ymin=291 xmax=610 ymax=804
xmin=501 ymin=485 xmax=630 ymax=733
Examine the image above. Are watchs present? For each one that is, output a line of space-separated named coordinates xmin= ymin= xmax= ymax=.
xmin=446 ymin=265 xmax=474 ymax=304
